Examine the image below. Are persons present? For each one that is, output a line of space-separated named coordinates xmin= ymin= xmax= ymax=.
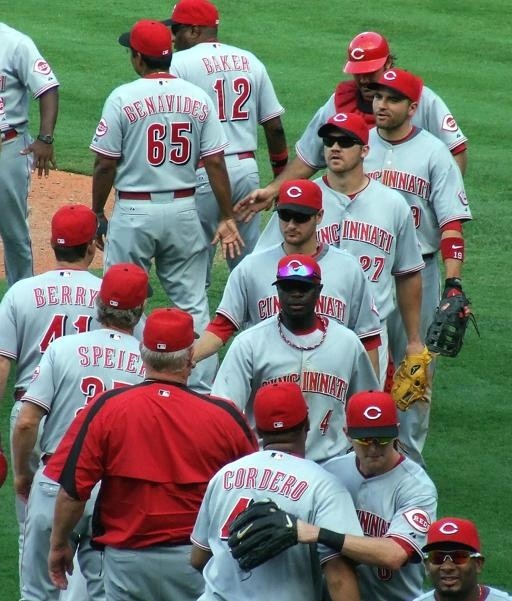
xmin=228 ymin=390 xmax=439 ymax=601
xmin=89 ymin=20 xmax=246 ymax=397
xmin=0 ymin=205 xmax=148 ymax=489
xmin=158 ymin=0 xmax=287 ymax=293
xmin=252 ymin=111 xmax=433 ymax=412
xmin=190 ymin=179 xmax=383 ymax=383
xmin=363 ymin=69 xmax=473 ymax=468
xmin=190 ymin=382 xmax=365 ymax=601
xmin=12 ymin=264 xmax=151 ymax=601
xmin=233 ymin=32 xmax=469 ymax=224
xmin=210 ymin=254 xmax=381 ymax=465
xmin=43 ymin=308 xmax=259 ymax=601
xmin=0 ymin=21 xmax=59 ymax=287
xmin=413 ymin=517 xmax=512 ymax=601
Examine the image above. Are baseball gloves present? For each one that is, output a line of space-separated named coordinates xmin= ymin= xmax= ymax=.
xmin=427 ymin=278 xmax=471 ymax=356
xmin=392 ymin=345 xmax=431 ymax=412
xmin=228 ymin=501 xmax=297 ymax=572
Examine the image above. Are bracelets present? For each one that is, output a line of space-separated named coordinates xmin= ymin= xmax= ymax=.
xmin=317 ymin=528 xmax=345 ymax=552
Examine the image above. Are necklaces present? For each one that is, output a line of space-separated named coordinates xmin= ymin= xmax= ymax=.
xmin=435 ymin=584 xmax=481 ymax=601
xmin=278 ymin=311 xmax=326 ymax=351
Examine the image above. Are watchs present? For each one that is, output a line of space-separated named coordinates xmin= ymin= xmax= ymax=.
xmin=37 ymin=135 xmax=54 ymax=144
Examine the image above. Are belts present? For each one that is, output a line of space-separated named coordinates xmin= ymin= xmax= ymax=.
xmin=0 ymin=127 xmax=18 ymax=142
xmin=196 ymin=150 xmax=255 ymax=170
xmin=42 ymin=453 xmax=52 ymax=467
xmin=117 ymin=185 xmax=197 ymax=200
xmin=14 ymin=390 xmax=27 ymax=403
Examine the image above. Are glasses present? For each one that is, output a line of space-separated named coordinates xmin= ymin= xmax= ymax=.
xmin=321 ymin=134 xmax=365 ymax=149
xmin=425 ymin=550 xmax=481 ymax=565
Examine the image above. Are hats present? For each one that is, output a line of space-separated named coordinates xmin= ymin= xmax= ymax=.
xmin=272 ymin=178 xmax=325 ymax=217
xmin=346 ymin=390 xmax=398 ymax=440
xmin=99 ymin=261 xmax=152 ymax=309
xmin=373 ymin=69 xmax=423 ymax=106
xmin=118 ymin=19 xmax=172 ymax=58
xmin=142 ymin=306 xmax=201 ymax=354
xmin=50 ymin=205 xmax=107 ymax=246
xmin=271 ymin=253 xmax=321 ymax=288
xmin=342 ymin=31 xmax=391 ymax=76
xmin=317 ymin=110 xmax=372 ymax=149
xmin=419 ymin=517 xmax=480 ymax=553
xmin=158 ymin=0 xmax=221 ymax=28
xmin=254 ymin=379 xmax=308 ymax=434
